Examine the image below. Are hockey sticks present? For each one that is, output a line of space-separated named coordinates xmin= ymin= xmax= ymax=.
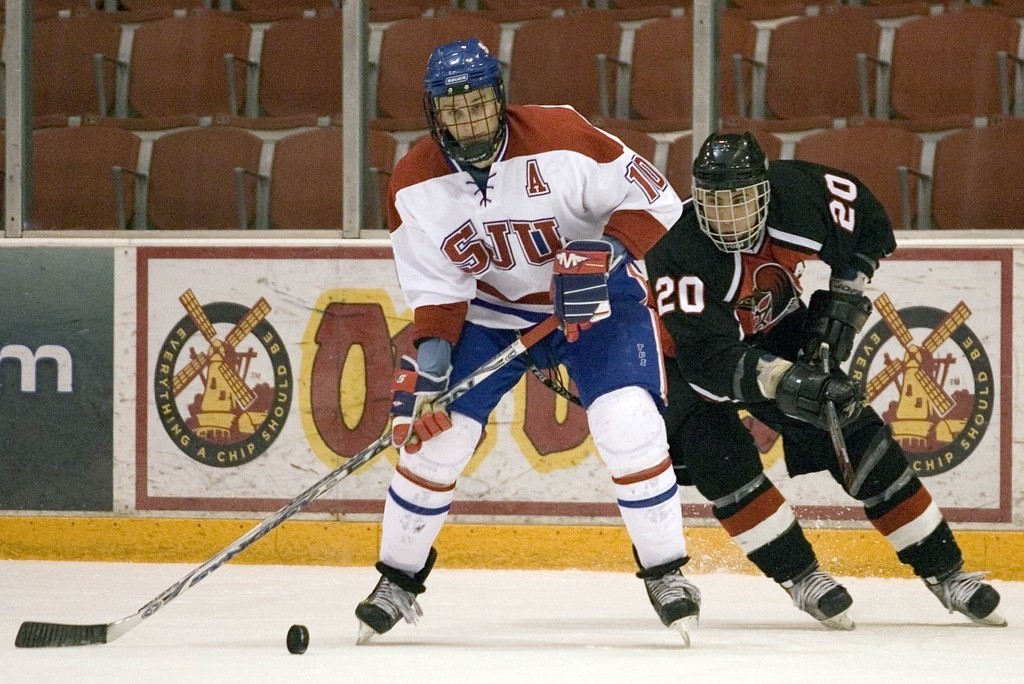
xmin=815 ymin=341 xmax=894 ymax=498
xmin=13 ymin=313 xmax=562 ymax=650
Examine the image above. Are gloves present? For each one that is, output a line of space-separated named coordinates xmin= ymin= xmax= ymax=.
xmin=390 ymin=355 xmax=452 ymax=453
xmin=551 ymin=239 xmax=613 ymax=343
xmin=795 ymin=289 xmax=873 ymax=370
xmin=776 ymin=363 xmax=863 ymax=432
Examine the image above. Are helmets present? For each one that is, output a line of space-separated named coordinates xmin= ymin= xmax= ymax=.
xmin=422 ymin=39 xmax=506 ymax=163
xmin=692 ymin=131 xmax=770 ymax=254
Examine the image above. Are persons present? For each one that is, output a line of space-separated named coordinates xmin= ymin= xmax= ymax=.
xmin=355 ymin=36 xmax=700 ymax=635
xmin=644 ymin=131 xmax=999 ymax=620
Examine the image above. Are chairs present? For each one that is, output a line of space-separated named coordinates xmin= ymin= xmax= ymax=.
xmin=1 ymin=0 xmax=1024 ymax=234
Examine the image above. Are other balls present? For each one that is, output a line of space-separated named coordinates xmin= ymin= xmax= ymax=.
xmin=283 ymin=624 xmax=311 ymax=655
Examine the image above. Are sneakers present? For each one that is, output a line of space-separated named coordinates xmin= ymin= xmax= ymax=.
xmin=777 ymin=562 xmax=854 ymax=631
xmin=632 ymin=543 xmax=701 ymax=648
xmin=920 ymin=562 xmax=1009 ymax=626
xmin=354 ymin=547 xmax=437 ymax=645
